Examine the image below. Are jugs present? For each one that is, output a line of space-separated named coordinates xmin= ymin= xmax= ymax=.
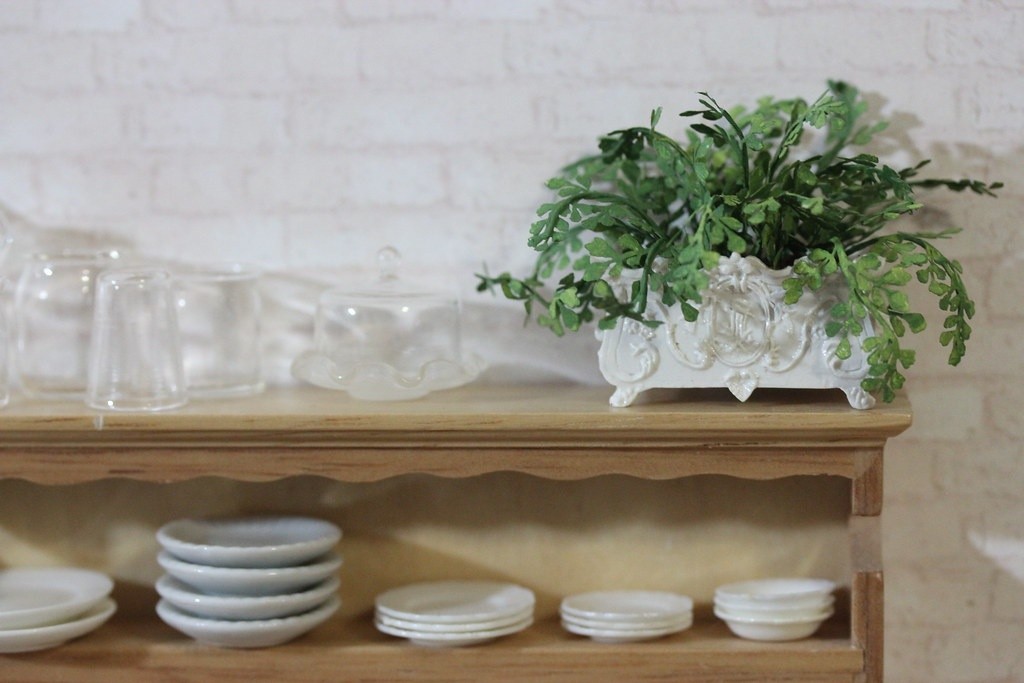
xmin=0 ymin=225 xmax=133 ymax=409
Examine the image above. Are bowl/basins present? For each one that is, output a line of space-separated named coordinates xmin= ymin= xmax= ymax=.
xmin=714 ymin=576 xmax=839 ymax=642
xmin=287 ymin=245 xmax=489 ymax=401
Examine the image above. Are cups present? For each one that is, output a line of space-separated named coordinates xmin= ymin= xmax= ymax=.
xmin=86 ymin=265 xmax=188 ymax=412
xmin=178 ymin=266 xmax=267 ymax=399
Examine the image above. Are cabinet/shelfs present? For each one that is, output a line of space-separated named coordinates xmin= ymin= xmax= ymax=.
xmin=0 ymin=378 xmax=912 ymax=683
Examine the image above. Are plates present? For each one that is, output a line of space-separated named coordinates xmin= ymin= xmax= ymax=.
xmin=559 ymin=588 xmax=695 ymax=643
xmin=0 ymin=564 xmax=118 ymax=654
xmin=372 ymin=578 xmax=537 ymax=649
xmin=153 ymin=516 xmax=346 ymax=650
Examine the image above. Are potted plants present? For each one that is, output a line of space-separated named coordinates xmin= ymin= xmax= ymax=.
xmin=472 ymin=77 xmax=1006 ymax=409
